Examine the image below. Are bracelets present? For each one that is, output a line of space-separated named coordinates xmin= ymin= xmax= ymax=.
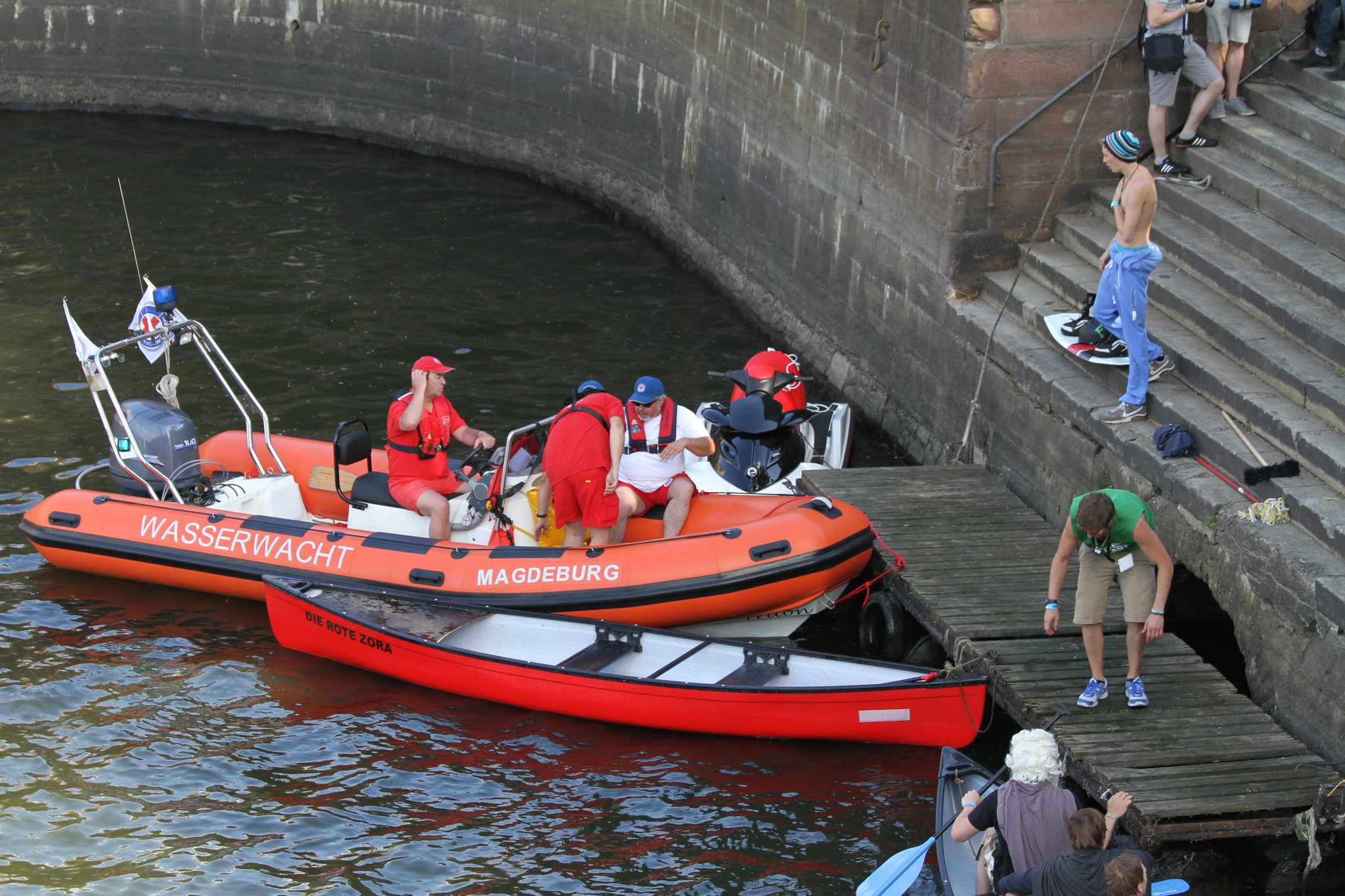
xmin=477 ymin=431 xmax=487 ymax=438
xmin=535 ymin=511 xmax=548 ymax=518
xmin=1110 ymin=201 xmax=1120 ymax=208
xmin=1045 ymin=603 xmax=1058 ymax=609
xmin=964 ymin=802 xmax=977 ymax=808
xmin=1151 ymin=609 xmax=1164 ymax=615
xmin=1104 ymin=814 xmax=1117 ymax=822
xmin=1184 ymin=5 xmax=1189 ymax=13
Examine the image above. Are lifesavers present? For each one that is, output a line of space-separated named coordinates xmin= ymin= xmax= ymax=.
xmin=860 ymin=589 xmax=904 ymax=658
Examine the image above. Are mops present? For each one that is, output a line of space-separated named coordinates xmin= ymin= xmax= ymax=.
xmin=1193 ymin=454 xmax=1293 ymax=527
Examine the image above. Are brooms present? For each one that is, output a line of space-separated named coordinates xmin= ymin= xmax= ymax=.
xmin=1220 ymin=406 xmax=1302 ymax=487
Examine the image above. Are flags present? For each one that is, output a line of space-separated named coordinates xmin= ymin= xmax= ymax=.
xmin=127 ymin=284 xmax=187 ymax=364
xmin=63 ymin=305 xmax=100 ymax=375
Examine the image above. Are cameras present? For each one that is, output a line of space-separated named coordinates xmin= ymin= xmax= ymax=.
xmin=1196 ymin=0 xmax=1216 ymax=7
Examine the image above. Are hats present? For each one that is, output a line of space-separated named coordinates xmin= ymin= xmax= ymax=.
xmin=412 ymin=356 xmax=455 ymax=374
xmin=1102 ymin=130 xmax=1140 ymax=163
xmin=627 ymin=376 xmax=665 ymax=404
xmin=578 ymin=380 xmax=604 ymax=393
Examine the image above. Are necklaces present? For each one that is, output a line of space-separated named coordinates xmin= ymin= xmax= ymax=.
xmin=1122 ymin=164 xmax=1139 ymax=192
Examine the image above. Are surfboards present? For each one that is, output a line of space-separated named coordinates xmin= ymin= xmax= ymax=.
xmin=1043 ymin=313 xmax=1129 ymax=365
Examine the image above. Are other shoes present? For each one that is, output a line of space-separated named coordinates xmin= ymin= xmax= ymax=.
xmin=1323 ymin=63 xmax=1345 ymax=81
xmin=1288 ymin=49 xmax=1332 ymax=68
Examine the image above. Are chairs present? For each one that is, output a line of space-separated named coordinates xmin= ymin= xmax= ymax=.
xmin=333 ymin=418 xmax=471 ymax=543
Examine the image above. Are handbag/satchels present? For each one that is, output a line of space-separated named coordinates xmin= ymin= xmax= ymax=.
xmin=1304 ymin=0 xmax=1344 ymax=51
xmin=1142 ymin=32 xmax=1186 ymax=74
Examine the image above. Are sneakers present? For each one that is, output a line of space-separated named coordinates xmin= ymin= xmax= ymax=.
xmin=1153 ymin=155 xmax=1192 ymax=174
xmin=1095 ymin=334 xmax=1129 ymax=357
xmin=1175 ymin=132 xmax=1218 ymax=147
xmin=1077 ymin=676 xmax=1110 ymax=708
xmin=1148 ymin=354 xmax=1176 ymax=383
xmin=1125 ymin=675 xmax=1150 ymax=707
xmin=1209 ymin=98 xmax=1226 ymax=120
xmin=1061 ymin=293 xmax=1096 ymax=337
xmin=1224 ymin=97 xmax=1257 ymax=116
xmin=1098 ymin=401 xmax=1147 ymax=424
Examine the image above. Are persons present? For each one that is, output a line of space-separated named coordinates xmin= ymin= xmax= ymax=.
xmin=999 ymin=807 xmax=1156 ymax=895
xmin=1093 ymin=130 xmax=1178 ymax=425
xmin=1288 ymin=0 xmax=1345 ymax=82
xmin=384 ymin=357 xmax=495 ymax=540
xmin=1142 ymin=0 xmax=1226 ymax=175
xmin=951 ymin=727 xmax=1133 ymax=896
xmin=1204 ymin=0 xmax=1257 ymax=120
xmin=1043 ymin=488 xmax=1174 ymax=708
xmin=608 ymin=375 xmax=715 ymax=545
xmin=534 ymin=380 xmax=626 ymax=548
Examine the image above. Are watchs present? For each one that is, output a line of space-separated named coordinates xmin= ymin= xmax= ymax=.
xmin=1045 ymin=597 xmax=1058 ymax=605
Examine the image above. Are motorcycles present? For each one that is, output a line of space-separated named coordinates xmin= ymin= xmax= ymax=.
xmin=694 ymin=345 xmax=858 ymax=494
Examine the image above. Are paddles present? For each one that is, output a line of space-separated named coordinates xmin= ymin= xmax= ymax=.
xmin=855 ymin=703 xmax=1073 ymax=896
xmin=1151 ymin=876 xmax=1189 ymax=896
xmin=881 ymin=672 xmax=938 ymax=683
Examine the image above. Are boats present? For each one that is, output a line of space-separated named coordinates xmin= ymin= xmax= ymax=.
xmin=17 ymin=297 xmax=881 ymax=642
xmin=931 ymin=742 xmax=1012 ymax=896
xmin=261 ymin=572 xmax=992 ymax=749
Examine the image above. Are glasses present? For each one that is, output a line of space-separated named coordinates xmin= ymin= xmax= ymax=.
xmin=632 ymin=400 xmax=657 ymax=408
xmin=1077 ymin=523 xmax=1104 ymax=537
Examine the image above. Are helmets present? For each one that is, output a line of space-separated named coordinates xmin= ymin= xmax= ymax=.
xmin=1077 ymin=319 xmax=1108 ymax=345
